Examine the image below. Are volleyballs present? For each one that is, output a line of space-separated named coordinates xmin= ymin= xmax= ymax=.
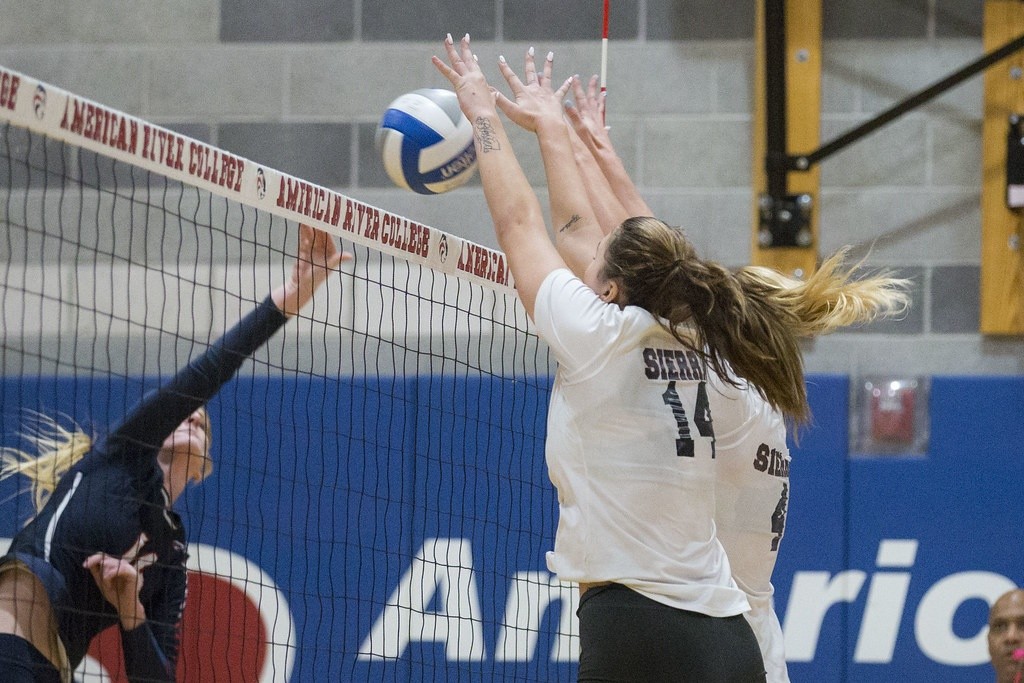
xmin=376 ymin=91 xmax=478 ymax=194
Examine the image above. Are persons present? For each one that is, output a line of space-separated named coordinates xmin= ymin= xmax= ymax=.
xmin=987 ymin=591 xmax=1024 ymax=683
xmin=430 ymin=31 xmax=809 ymax=683
xmin=563 ymin=71 xmax=904 ymax=683
xmin=0 ymin=219 xmax=352 ymax=683
